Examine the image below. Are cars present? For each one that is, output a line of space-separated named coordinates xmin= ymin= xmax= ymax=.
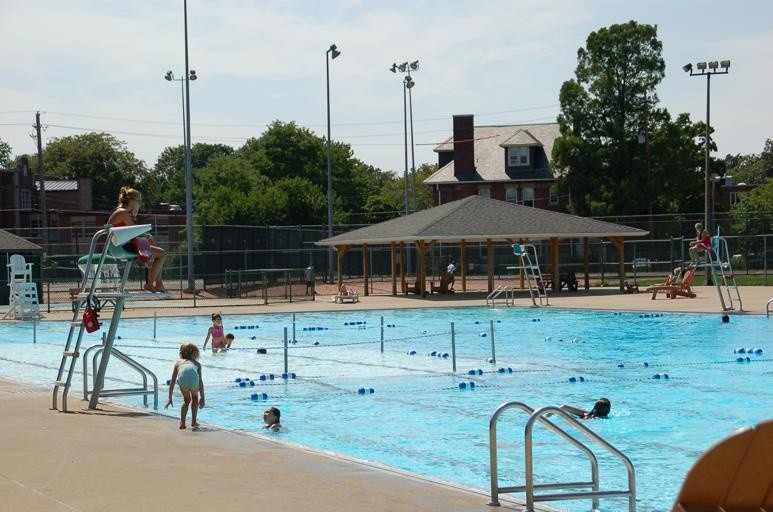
xmin=632 ymin=258 xmax=652 ymax=271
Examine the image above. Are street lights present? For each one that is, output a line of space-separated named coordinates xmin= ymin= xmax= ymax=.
xmin=164 ymin=69 xmax=198 ymax=293
xmin=682 ymin=60 xmax=730 ymax=286
xmin=326 ymin=44 xmax=341 ymax=284
xmin=389 ymin=59 xmax=420 ymax=276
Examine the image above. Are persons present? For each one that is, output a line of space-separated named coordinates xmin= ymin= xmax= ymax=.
xmin=687 ymin=222 xmax=711 ymax=268
xmin=223 ymin=334 xmax=234 ymax=350
xmin=202 ymin=312 xmax=225 ymax=354
xmin=543 ymin=398 xmax=610 ymax=420
xmin=233 ymin=407 xmax=289 ymax=433
xmin=437 ymin=267 xmax=451 ymax=296
xmin=338 ymin=283 xmax=354 ymax=296
xmin=106 ymin=186 xmax=168 ymax=294
xmin=649 ymin=267 xmax=681 ymax=287
xmin=162 ymin=342 xmax=206 ymax=429
xmin=622 ymin=281 xmax=639 ymax=293
xmin=446 ymin=260 xmax=456 ymax=291
xmin=303 ymin=263 xmax=316 ymax=296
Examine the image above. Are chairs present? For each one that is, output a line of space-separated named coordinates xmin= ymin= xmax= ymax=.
xmin=7 ymin=254 xmax=35 ymax=283
xmin=692 ymin=237 xmax=719 ymax=264
xmin=74 ymin=223 xmax=142 ymax=291
xmin=334 ymin=294 xmax=359 ymax=304
xmin=647 ymin=271 xmax=697 ymax=299
xmin=511 ymin=242 xmax=526 ymax=256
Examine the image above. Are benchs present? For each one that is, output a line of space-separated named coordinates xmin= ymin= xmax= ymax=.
xmin=537 ymin=275 xmax=580 ymax=291
xmin=69 ymin=286 xmax=125 ymax=311
